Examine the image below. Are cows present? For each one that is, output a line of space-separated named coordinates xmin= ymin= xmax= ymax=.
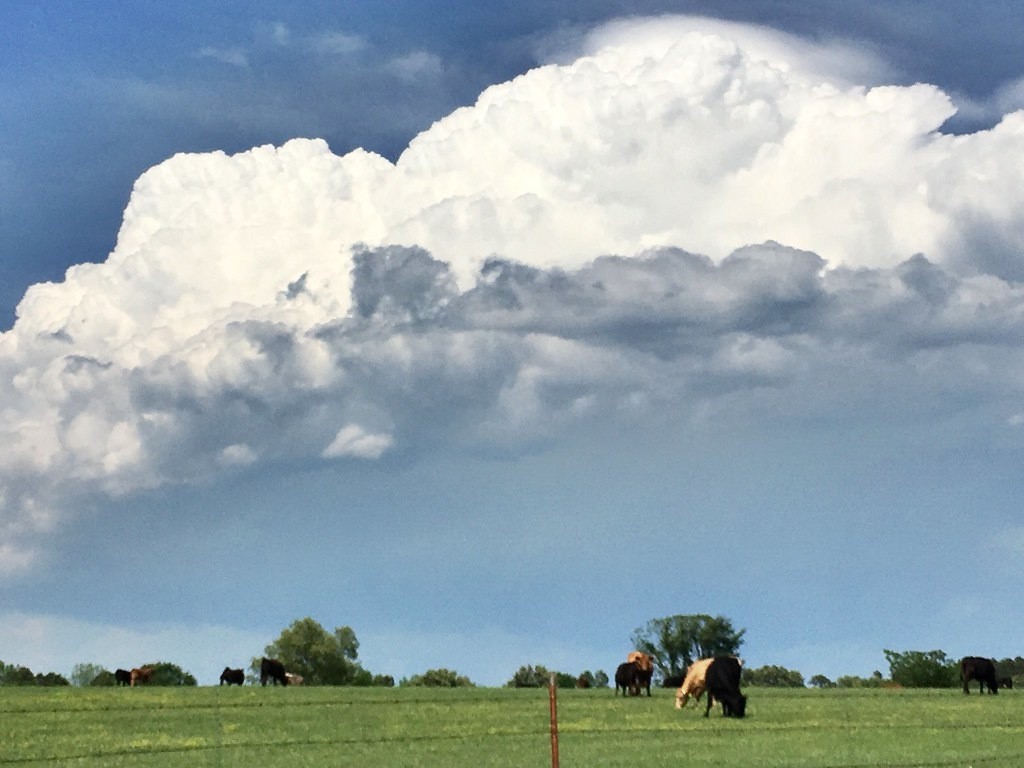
xmin=114 ymin=669 xmax=131 ymax=687
xmin=129 ymin=667 xmax=151 ymax=686
xmin=614 ymin=661 xmax=652 ymax=698
xmin=959 ymin=656 xmax=1000 ymax=694
xmin=259 ymin=658 xmax=290 ymax=687
xmin=703 ymin=656 xmax=747 ymax=718
xmin=628 ymin=651 xmax=655 ymax=689
xmin=220 ymin=667 xmax=245 ymax=687
xmin=675 ymin=656 xmax=742 ymax=709
